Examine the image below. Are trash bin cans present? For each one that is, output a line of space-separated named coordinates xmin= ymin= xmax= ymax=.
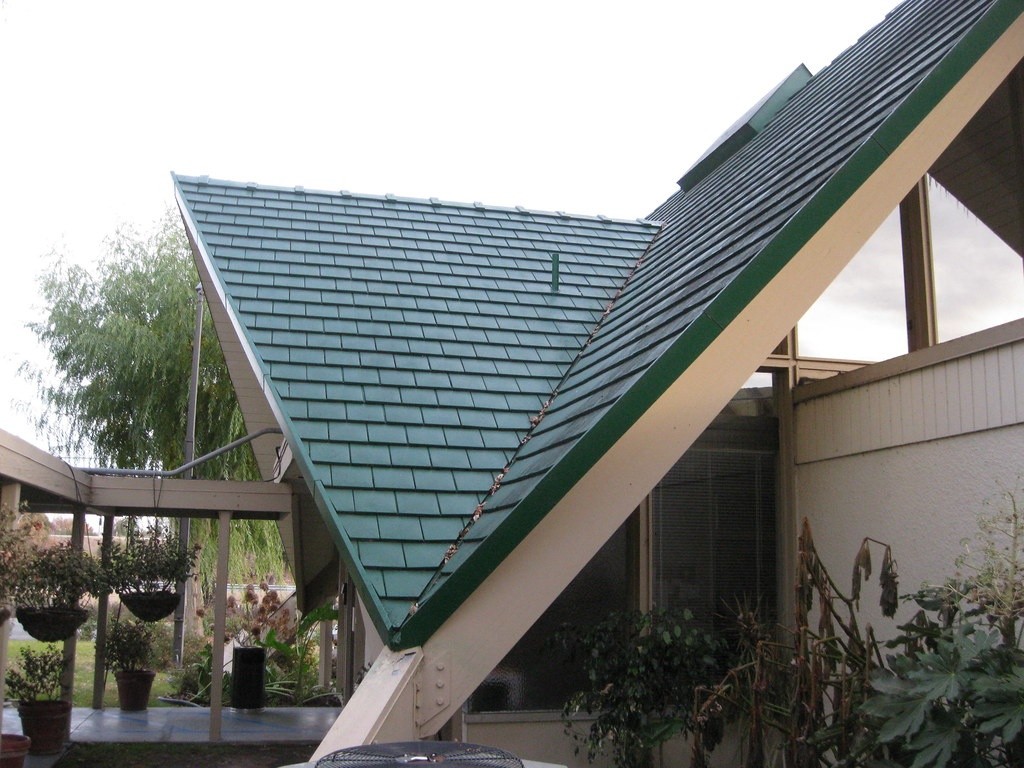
xmin=230 ymin=647 xmax=266 ymax=713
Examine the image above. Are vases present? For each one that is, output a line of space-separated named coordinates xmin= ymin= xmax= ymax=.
xmin=230 ymin=648 xmax=265 ymax=709
xmin=119 ymin=592 xmax=181 ymax=622
xmin=0 ymin=733 xmax=31 ymax=768
xmin=16 ymin=606 xmax=88 ymax=643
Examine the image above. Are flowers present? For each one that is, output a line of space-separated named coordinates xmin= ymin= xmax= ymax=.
xmin=196 ymin=580 xmax=296 ymax=646
xmin=0 ymin=497 xmax=204 ymax=608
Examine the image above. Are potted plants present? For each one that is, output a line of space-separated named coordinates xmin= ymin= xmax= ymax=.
xmin=5 ymin=642 xmax=72 ymax=756
xmin=94 ymin=617 xmax=156 ymax=713
